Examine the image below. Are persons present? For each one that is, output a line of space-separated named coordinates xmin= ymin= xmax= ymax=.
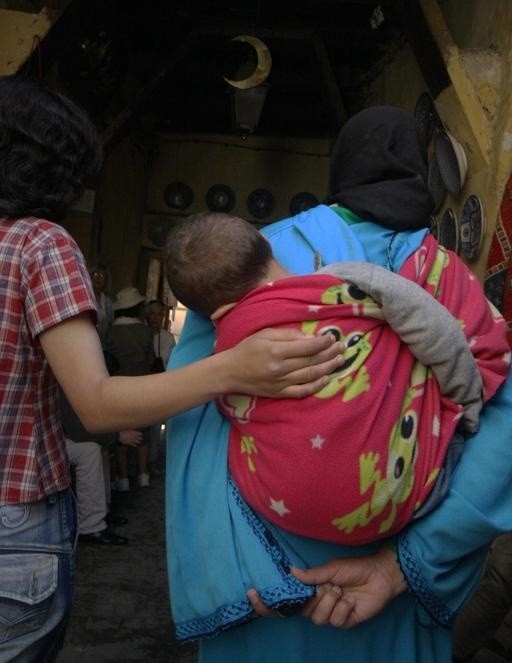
xmin=164 ymin=213 xmax=486 ymax=545
xmin=0 ymin=75 xmax=346 ymax=663
xmin=60 ymin=263 xmax=176 ymax=544
xmin=163 ymin=106 xmax=512 ymax=662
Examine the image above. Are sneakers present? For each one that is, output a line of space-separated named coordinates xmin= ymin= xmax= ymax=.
xmin=80 ymin=474 xmax=150 ymax=546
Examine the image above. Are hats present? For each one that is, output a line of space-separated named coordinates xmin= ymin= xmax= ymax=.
xmin=112 ymin=288 xmax=144 ymax=310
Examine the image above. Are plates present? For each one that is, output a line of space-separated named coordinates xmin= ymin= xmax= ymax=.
xmin=426 ymin=122 xmax=469 ymax=196
xmin=410 ymin=90 xmax=446 ymax=210
xmin=161 ymin=181 xmax=320 ymax=219
xmin=458 ymin=191 xmax=485 ymax=259
xmin=439 ymin=207 xmax=458 ymax=254
xmin=424 ymin=215 xmax=437 ymax=242
xmin=142 ymin=218 xmax=177 ymax=247
